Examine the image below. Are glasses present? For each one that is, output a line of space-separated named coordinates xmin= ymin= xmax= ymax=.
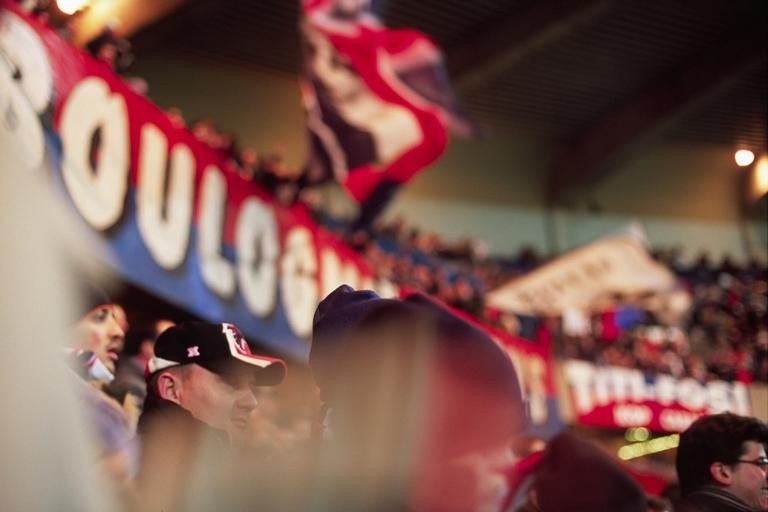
xmin=725 ymin=456 xmax=767 ymax=470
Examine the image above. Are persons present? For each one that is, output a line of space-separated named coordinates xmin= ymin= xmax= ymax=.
xmin=1 ymin=0 xmax=768 ymax=511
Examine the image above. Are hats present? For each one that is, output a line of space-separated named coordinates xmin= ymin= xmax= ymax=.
xmin=308 ymin=285 xmax=526 ymax=452
xmin=534 ymin=433 xmax=648 ymax=512
xmin=70 ymin=279 xmax=112 ymax=327
xmin=144 ymin=321 xmax=286 ymax=385
